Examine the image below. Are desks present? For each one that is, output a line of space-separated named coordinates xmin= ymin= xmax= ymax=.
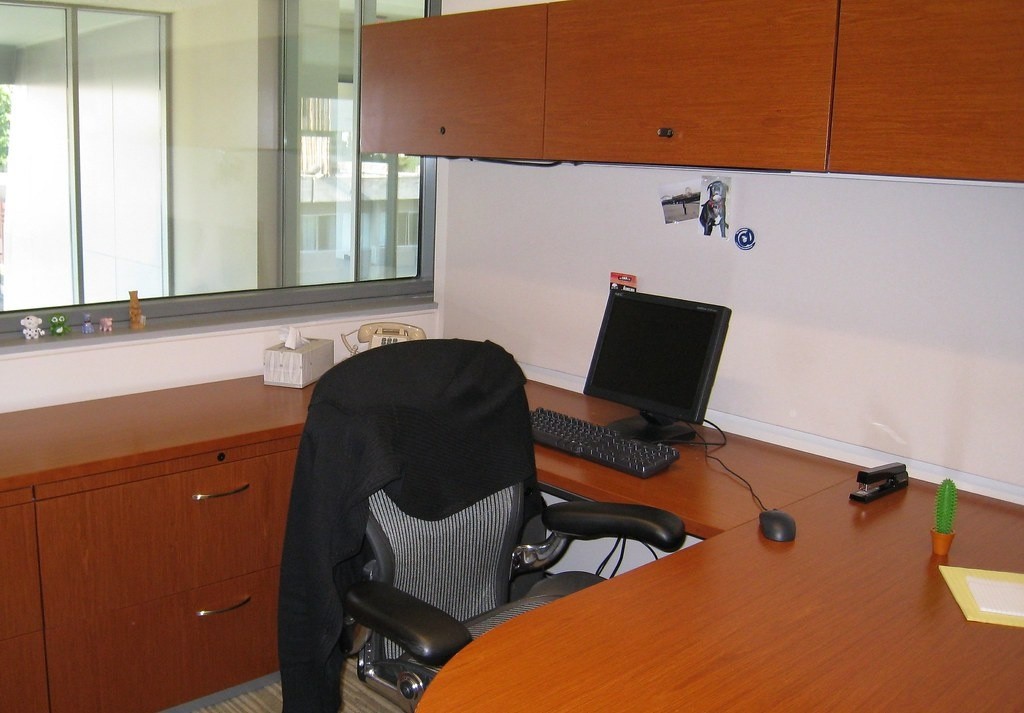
xmin=411 ymin=461 xmax=1024 ymax=713
xmin=518 ymin=379 xmax=875 ymax=542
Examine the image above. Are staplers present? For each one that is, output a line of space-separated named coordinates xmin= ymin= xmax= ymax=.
xmin=849 ymin=462 xmax=909 ymax=504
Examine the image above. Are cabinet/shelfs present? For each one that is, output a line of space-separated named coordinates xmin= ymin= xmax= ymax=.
xmin=360 ymin=0 xmax=1024 ymax=184
xmin=0 ymin=375 xmax=317 ymax=713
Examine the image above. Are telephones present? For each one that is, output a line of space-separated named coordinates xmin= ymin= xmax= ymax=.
xmin=342 ymin=322 xmax=427 ymax=357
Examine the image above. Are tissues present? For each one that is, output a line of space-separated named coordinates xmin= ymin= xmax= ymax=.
xmin=263 ymin=327 xmax=334 ymax=389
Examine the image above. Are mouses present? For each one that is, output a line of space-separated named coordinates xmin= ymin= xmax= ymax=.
xmin=759 ymin=508 xmax=796 ymax=541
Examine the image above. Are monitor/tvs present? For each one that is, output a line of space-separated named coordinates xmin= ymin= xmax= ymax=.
xmin=583 ymin=288 xmax=732 ymax=445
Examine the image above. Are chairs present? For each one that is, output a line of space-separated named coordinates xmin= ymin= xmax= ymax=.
xmin=276 ymin=338 xmax=685 ymax=713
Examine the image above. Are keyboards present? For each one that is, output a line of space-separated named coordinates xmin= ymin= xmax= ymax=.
xmin=529 ymin=407 xmax=680 ymax=479
